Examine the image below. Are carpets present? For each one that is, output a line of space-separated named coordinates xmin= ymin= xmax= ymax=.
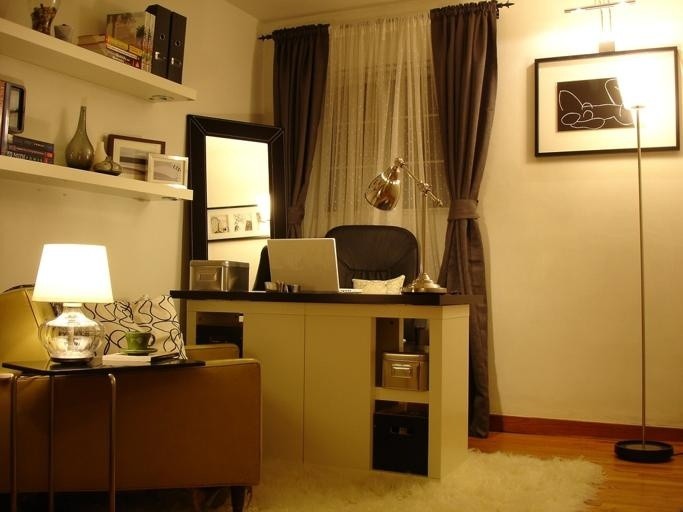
xmin=242 ymin=449 xmax=605 ymax=512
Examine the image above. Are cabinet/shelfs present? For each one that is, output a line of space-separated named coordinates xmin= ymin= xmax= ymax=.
xmin=168 ymin=287 xmax=486 ymax=479
xmin=0 ymin=19 xmax=200 ymax=200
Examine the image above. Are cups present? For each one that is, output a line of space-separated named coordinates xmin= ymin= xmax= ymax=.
xmin=124 ymin=331 xmax=155 ymax=350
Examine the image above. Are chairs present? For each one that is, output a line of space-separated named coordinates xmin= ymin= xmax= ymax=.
xmin=324 ymin=225 xmax=419 ymax=297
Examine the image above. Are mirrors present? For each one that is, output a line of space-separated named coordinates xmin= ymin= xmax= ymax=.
xmin=186 ymin=114 xmax=289 ymax=291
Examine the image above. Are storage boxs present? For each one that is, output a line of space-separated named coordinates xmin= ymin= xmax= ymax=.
xmin=383 ymin=352 xmax=428 ymax=389
xmin=371 ymin=412 xmax=428 ymax=475
xmin=188 ymin=257 xmax=249 ymax=292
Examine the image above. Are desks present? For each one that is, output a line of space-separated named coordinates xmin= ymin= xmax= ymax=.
xmin=5 ymin=360 xmax=207 ymax=511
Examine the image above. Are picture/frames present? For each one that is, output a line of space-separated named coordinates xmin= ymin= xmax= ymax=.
xmin=207 ymin=204 xmax=270 ymax=241
xmin=108 ymin=134 xmax=165 ymax=181
xmin=534 ymin=46 xmax=679 ymax=157
xmin=146 ymin=153 xmax=189 ymax=186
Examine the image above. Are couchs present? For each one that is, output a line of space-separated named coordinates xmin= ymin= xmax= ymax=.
xmin=0 ymin=343 xmax=263 ymax=511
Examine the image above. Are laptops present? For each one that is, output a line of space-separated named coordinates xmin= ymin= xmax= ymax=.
xmin=267 ymin=237 xmax=365 ymax=294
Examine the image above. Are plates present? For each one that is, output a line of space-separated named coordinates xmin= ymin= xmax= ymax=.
xmin=118 ymin=349 xmax=157 ymax=356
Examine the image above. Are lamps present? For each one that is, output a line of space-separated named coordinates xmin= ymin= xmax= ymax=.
xmin=31 ymin=243 xmax=116 ymax=364
xmin=363 ymin=157 xmax=449 ymax=292
xmin=615 ymin=66 xmax=677 ymax=467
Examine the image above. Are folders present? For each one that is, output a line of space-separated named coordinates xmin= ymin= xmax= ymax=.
xmin=144 ymin=5 xmax=171 ymax=81
xmin=168 ymin=12 xmax=187 ymax=86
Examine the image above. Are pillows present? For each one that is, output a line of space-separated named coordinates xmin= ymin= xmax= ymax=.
xmin=78 ymin=295 xmax=192 ymax=362
xmin=353 ymin=273 xmax=405 ymax=295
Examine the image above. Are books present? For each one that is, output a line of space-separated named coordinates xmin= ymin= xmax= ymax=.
xmin=76 ymin=10 xmax=156 ymax=73
xmin=7 ymin=144 xmax=54 ymax=158
xmin=6 ymin=151 xmax=54 ymax=164
xmin=102 ymin=352 xmax=179 ymax=362
xmin=7 ymin=133 xmax=54 ymax=152
